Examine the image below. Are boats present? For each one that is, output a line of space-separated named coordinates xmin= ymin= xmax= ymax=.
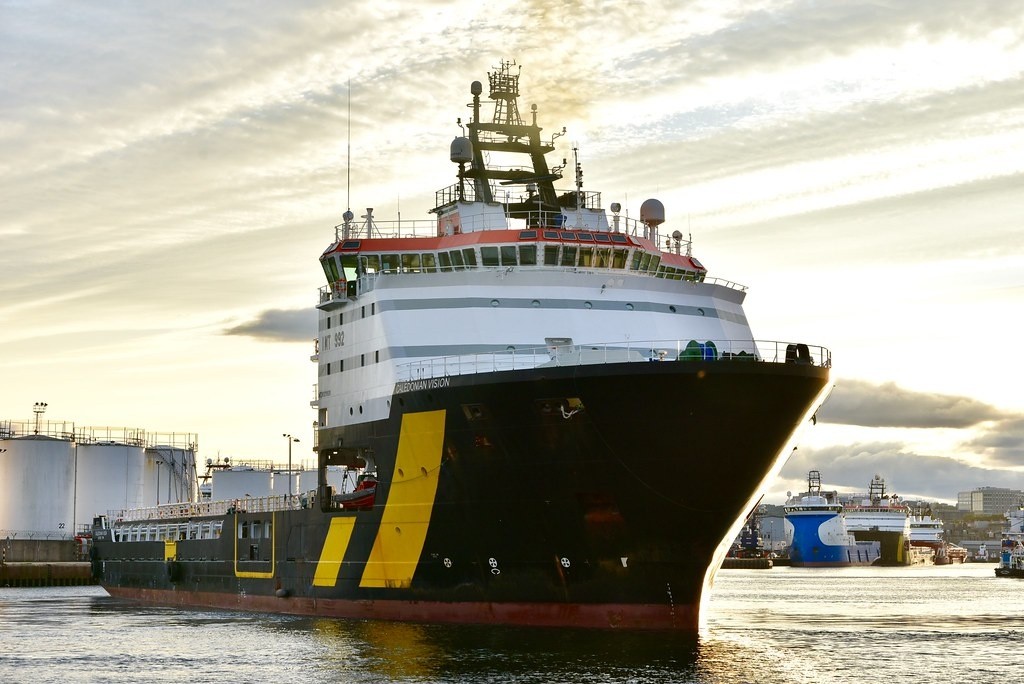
xmin=71 ymin=58 xmax=833 ymax=634
xmin=720 ymin=470 xmax=1001 ymax=570
xmin=993 ymin=500 xmax=1024 ymax=579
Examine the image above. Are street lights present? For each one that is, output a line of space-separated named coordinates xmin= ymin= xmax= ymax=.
xmin=282 ymin=434 xmax=300 ymax=494
